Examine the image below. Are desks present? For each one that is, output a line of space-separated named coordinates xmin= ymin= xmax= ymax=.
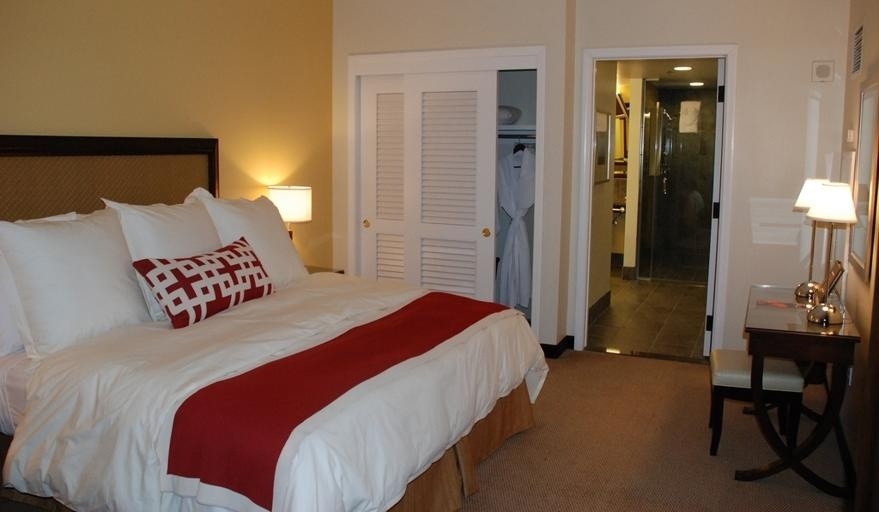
xmin=733 ymin=285 xmax=860 ymax=502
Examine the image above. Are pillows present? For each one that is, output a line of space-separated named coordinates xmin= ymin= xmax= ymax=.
xmin=98 ymin=186 xmax=223 ymax=322
xmin=199 ymin=196 xmax=309 ymax=294
xmin=1 ymin=210 xmax=90 ymax=356
xmin=1 ymin=208 xmax=153 ymax=356
xmin=132 ymin=236 xmax=274 ymax=329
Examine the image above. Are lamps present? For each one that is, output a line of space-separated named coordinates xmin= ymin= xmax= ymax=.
xmin=791 ymin=176 xmax=830 ymax=303
xmin=804 ymin=183 xmax=859 ymax=327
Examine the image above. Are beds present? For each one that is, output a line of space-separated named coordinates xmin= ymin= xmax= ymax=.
xmin=0 ymin=135 xmax=550 ymax=512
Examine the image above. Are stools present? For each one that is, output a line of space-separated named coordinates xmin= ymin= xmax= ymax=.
xmin=706 ymin=347 xmax=804 ymax=457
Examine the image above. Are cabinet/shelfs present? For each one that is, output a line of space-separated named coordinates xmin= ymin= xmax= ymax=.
xmin=344 ymin=44 xmax=544 ymax=359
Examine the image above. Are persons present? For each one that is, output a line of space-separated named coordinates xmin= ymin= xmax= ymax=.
xmin=665 ymin=174 xmax=703 ymax=235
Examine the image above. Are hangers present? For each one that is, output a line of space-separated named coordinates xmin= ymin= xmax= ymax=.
xmin=511 ymin=136 xmax=526 ymax=153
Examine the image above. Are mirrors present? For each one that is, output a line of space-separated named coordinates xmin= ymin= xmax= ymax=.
xmin=847 ymin=72 xmax=878 ymax=285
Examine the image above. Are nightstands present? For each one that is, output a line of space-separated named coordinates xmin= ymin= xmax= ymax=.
xmin=303 ymin=265 xmax=345 ymax=275
